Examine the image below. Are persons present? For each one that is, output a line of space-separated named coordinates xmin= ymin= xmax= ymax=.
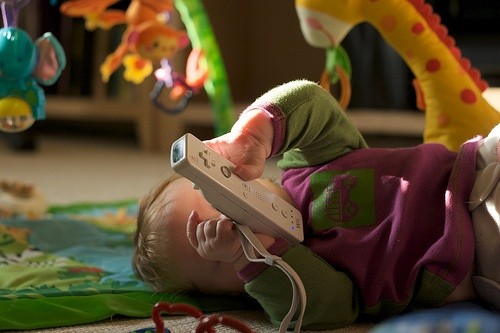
xmin=132 ymin=80 xmax=500 ymax=330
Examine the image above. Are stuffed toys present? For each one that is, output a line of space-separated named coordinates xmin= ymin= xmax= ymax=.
xmin=295 ymin=0 xmax=499 ymax=154
xmin=0 ymin=0 xmax=67 ymax=132
xmin=59 ymin=0 xmax=236 ymax=138
